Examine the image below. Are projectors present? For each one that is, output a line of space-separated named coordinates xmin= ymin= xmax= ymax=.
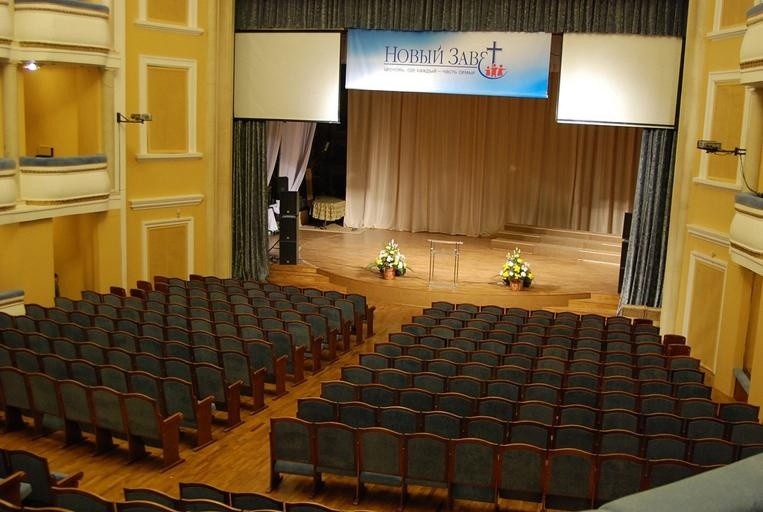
xmin=131 ymin=112 xmax=153 ymax=122
xmin=698 ymin=140 xmax=722 ymax=152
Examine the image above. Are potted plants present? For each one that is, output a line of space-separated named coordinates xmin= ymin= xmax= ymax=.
xmin=507 ymin=280 xmax=525 ymax=292
xmin=379 ymin=266 xmax=396 ymax=280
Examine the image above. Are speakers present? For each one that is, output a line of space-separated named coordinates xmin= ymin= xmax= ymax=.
xmin=618 ymin=213 xmax=633 ymax=292
xmin=278 ymin=191 xmax=299 ymax=265
xmin=276 ymin=176 xmax=288 ymax=201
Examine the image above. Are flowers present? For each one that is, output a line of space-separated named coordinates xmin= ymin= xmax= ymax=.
xmin=496 ymin=245 xmax=537 ymax=290
xmin=364 ymin=239 xmax=414 ymax=277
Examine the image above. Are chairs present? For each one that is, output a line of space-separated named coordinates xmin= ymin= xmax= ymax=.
xmin=50 ymin=484 xmax=114 ymax=512
xmin=285 ymin=500 xmax=339 ymax=512
xmin=8 ymin=448 xmax=85 ymax=503
xmin=122 ymin=486 xmax=180 ymax=509
xmin=230 ymin=492 xmax=284 ymax=511
xmin=177 ymin=481 xmax=230 ymax=507
xmin=116 ymin=500 xmax=177 ymax=512
xmin=0 ymin=469 xmax=29 ymax=505
xmin=180 ymin=497 xmax=241 ymax=512
